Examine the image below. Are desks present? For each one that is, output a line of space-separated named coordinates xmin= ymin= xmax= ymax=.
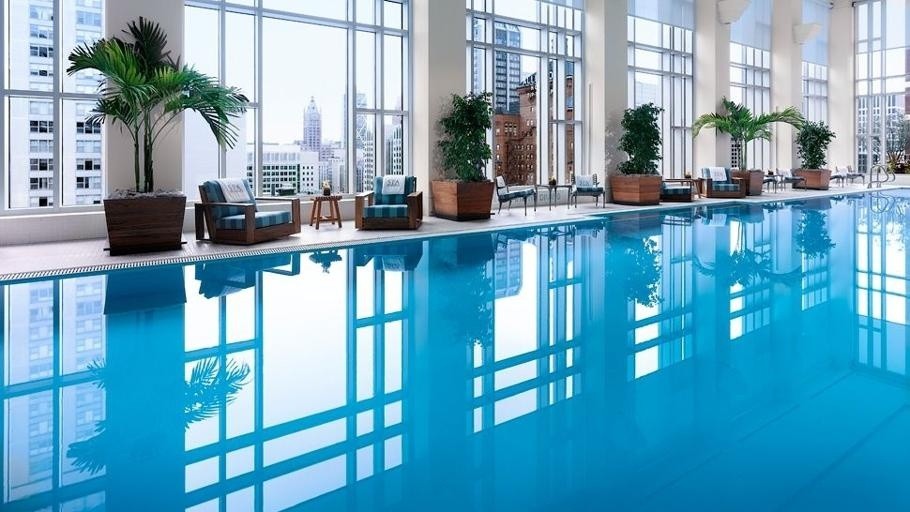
xmin=308 ymin=246 xmax=341 ymax=271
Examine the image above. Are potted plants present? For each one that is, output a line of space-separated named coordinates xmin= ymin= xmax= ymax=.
xmin=609 ymin=215 xmax=669 ymax=310
xmin=688 ymin=205 xmax=807 ymax=292
xmin=431 ymin=89 xmax=499 ymax=222
xmin=429 ymin=234 xmax=498 ymax=353
xmin=67 ymin=264 xmax=252 ymax=484
xmin=790 ymin=121 xmax=837 ymax=189
xmin=67 ymin=17 xmax=253 ymax=257
xmin=689 ymin=96 xmax=809 ymax=196
xmin=794 ymin=197 xmax=835 ymax=257
xmin=610 ymin=102 xmax=667 ymax=206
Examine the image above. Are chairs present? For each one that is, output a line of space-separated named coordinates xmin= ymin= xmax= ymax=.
xmin=354 ymin=241 xmax=424 ymax=272
xmin=193 ymin=251 xmax=301 ymax=300
